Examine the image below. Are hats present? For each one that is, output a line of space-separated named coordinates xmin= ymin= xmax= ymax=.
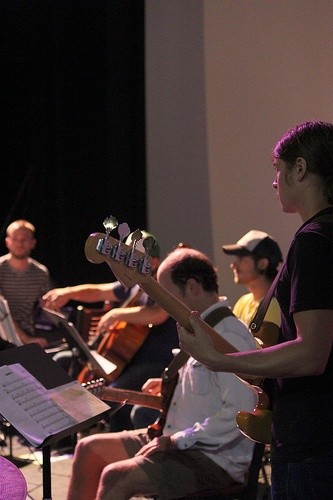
xmin=125 ymin=230 xmax=160 ymax=261
xmin=221 ymin=229 xmax=284 ymax=265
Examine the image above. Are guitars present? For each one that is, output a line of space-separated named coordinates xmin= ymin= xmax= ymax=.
xmin=84 ymin=214 xmax=282 ymax=443
xmin=81 ymin=377 xmax=178 ymax=440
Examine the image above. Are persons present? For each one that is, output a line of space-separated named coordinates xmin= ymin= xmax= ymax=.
xmin=66 ymin=248 xmax=262 ymax=500
xmin=176 ymin=122 xmax=333 ymax=500
xmin=130 ymin=229 xmax=284 ymax=429
xmin=0 ymin=220 xmax=62 ymax=349
xmin=42 ymin=231 xmax=182 ymax=456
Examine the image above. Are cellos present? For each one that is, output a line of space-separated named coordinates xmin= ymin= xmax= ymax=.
xmin=75 ymin=242 xmax=188 ymax=393
